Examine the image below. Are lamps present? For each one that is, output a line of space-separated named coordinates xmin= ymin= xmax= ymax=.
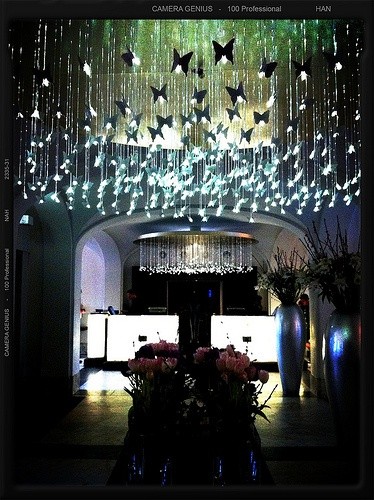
xmin=132 ymin=225 xmax=259 ymax=275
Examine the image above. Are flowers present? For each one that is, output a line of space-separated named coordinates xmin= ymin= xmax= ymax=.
xmin=127 ymin=339 xmax=268 ymax=422
xmin=251 ymin=214 xmax=361 ymax=309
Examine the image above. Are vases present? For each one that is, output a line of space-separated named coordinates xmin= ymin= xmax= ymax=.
xmin=275 ymin=303 xmax=307 ymax=398
xmin=319 ymin=310 xmax=357 ymax=403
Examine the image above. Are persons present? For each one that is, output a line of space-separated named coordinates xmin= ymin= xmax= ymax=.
xmin=299 ymin=294 xmax=310 ymax=372
xmin=121 ymin=289 xmax=149 ymax=315
